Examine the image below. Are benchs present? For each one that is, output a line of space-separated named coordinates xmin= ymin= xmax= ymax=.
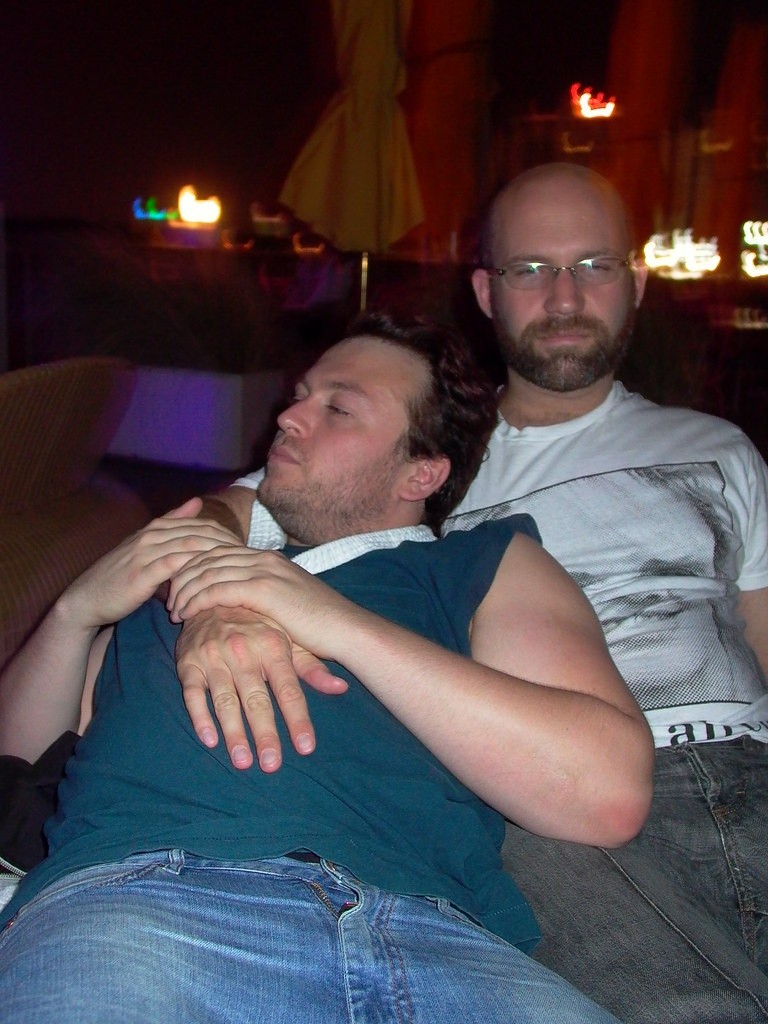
xmin=0 ymin=355 xmax=148 ymax=675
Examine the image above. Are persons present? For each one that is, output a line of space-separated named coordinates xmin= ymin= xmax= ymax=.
xmin=158 ymin=160 xmax=768 ymax=1024
xmin=3 ymin=309 xmax=658 ymax=1024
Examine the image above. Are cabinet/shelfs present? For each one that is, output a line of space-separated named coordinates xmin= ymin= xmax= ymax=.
xmin=4 ymin=230 xmax=324 ymax=371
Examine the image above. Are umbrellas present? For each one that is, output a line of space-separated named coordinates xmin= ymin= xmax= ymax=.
xmin=279 ymin=0 xmax=427 ymax=315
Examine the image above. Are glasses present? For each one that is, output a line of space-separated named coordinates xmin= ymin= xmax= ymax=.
xmin=491 ymin=256 xmax=634 ymax=289
